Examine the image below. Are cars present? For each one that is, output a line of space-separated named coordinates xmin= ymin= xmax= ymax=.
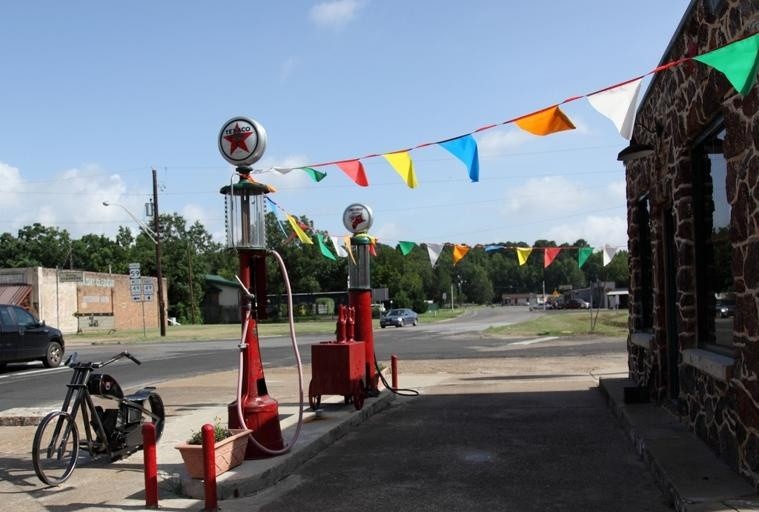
xmin=0 ymin=304 xmax=64 ymax=367
xmin=530 ymin=302 xmax=553 ymax=309
xmin=565 ymin=299 xmax=590 ymax=309
xmin=380 ymin=309 xmax=419 ymax=328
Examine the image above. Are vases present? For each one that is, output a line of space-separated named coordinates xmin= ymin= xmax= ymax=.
xmin=174 ymin=428 xmax=256 ymax=479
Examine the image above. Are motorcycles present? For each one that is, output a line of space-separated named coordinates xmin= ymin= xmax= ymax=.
xmin=30 ymin=350 xmax=165 ymax=487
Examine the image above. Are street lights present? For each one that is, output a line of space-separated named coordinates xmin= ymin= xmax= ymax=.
xmin=103 ymin=170 xmax=165 ymax=337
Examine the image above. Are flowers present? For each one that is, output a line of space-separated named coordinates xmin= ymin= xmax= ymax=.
xmin=188 ymin=414 xmax=235 ymax=442
